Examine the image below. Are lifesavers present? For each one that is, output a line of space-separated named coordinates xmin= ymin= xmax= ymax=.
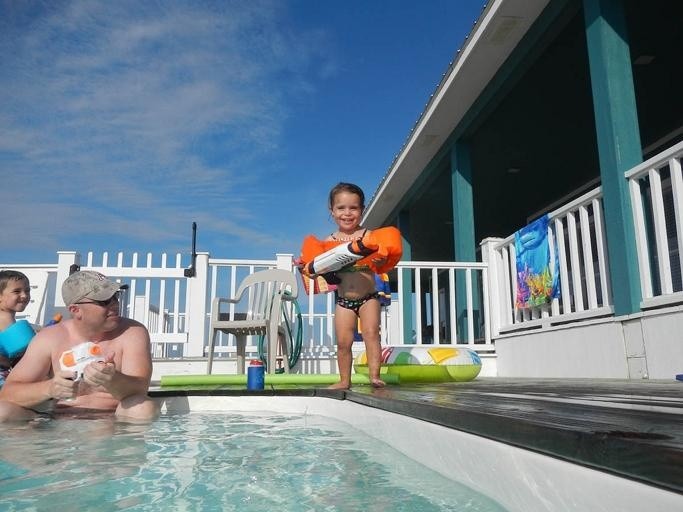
xmin=353 ymin=347 xmax=482 ymax=383
xmin=0 ymin=319 xmax=36 ymax=358
xmin=301 ymin=226 xmax=403 ymax=274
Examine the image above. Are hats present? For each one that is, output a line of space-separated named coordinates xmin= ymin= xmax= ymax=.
xmin=61 ymin=270 xmax=129 ymax=307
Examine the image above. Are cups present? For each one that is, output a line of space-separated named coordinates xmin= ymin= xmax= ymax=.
xmin=248 ymin=365 xmax=264 ymax=390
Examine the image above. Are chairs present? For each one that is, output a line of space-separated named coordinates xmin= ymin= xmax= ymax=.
xmin=207 ymin=268 xmax=299 ymax=374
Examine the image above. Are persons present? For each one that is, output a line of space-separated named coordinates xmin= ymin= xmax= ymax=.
xmin=298 ymin=182 xmax=387 ymax=390
xmin=0 ymin=270 xmax=159 ymax=425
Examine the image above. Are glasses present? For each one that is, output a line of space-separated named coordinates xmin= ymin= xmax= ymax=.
xmin=73 ymin=290 xmax=120 ymax=306
